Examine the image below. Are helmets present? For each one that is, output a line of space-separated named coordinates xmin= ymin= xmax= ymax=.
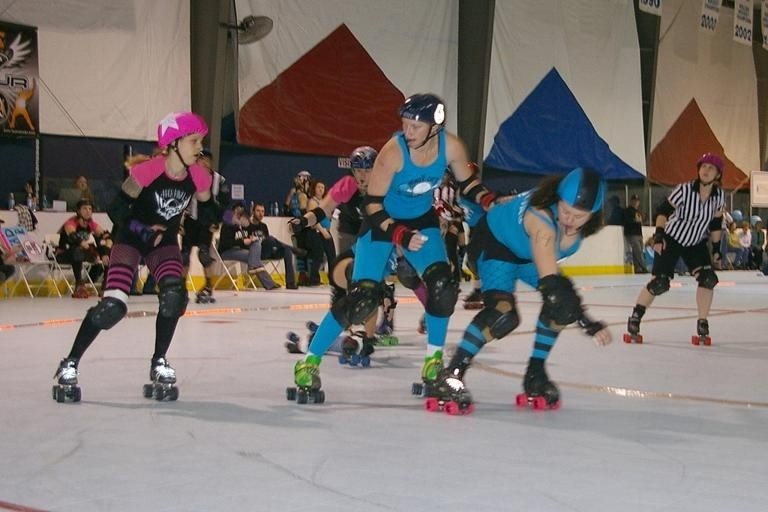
xmin=557 ymin=167 xmax=606 ymax=213
xmin=205 ymin=147 xmax=213 ymax=157
xmin=397 ymin=93 xmax=448 ymax=127
xmin=698 ymin=154 xmax=725 ymax=173
xmin=76 ymin=198 xmax=96 ymax=207
xmin=297 ymin=171 xmax=313 ymax=182
xmin=158 ymin=112 xmax=209 ymax=149
xmin=466 ymin=162 xmax=481 ymax=174
xmin=348 ymin=146 xmax=377 ymax=169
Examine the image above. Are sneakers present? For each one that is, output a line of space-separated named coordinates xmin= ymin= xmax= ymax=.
xmin=248 ymin=266 xmax=265 ymax=273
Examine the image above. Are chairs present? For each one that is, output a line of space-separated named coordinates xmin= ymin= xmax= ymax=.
xmin=10 ymin=260 xmax=63 ymax=300
xmin=211 ymin=239 xmax=258 ymax=291
xmin=45 ymin=233 xmax=100 ymax=297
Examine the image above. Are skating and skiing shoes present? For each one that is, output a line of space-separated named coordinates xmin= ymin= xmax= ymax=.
xmin=197 ymin=286 xmax=215 ymax=303
xmin=464 ymin=287 xmax=485 ymax=312
xmin=73 ymin=281 xmax=89 ymax=299
xmin=623 ymin=316 xmax=644 ymax=343
xmin=516 ymin=372 xmax=559 ymax=411
xmin=423 ymin=371 xmax=475 ymax=415
xmin=338 ymin=330 xmax=377 ymax=368
xmin=286 ymin=357 xmax=325 ymax=404
xmin=52 ymin=358 xmax=81 ymax=402
xmin=376 ymin=321 xmax=400 ymax=347
xmin=691 ymin=318 xmax=713 ymax=346
xmin=141 ymin=356 xmax=178 ymax=401
xmin=411 ymin=350 xmax=444 ymax=396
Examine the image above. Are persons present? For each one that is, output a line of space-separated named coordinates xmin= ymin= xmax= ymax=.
xmin=739 ymin=222 xmax=751 ymax=270
xmin=423 ymin=167 xmax=615 ymax=414
xmin=75 ymin=176 xmax=99 ymax=211
xmin=311 ymin=180 xmax=340 ymax=277
xmin=50 ymin=113 xmax=218 ymax=403
xmin=224 ymin=197 xmax=281 ymax=291
xmin=46 ymin=200 xmax=113 ymax=299
xmin=607 ymin=195 xmax=625 ymax=226
xmin=285 ymin=94 xmax=513 ymax=405
xmin=623 ymin=153 xmax=725 ymax=345
xmin=653 ymin=214 xmax=667 ymax=261
xmin=287 ymin=170 xmax=316 ymax=282
xmin=728 ymin=220 xmax=745 ymax=270
xmin=286 ymin=147 xmax=436 ymax=354
xmin=0 ymin=219 xmax=22 ymax=287
xmin=182 ymin=147 xmax=229 ymax=304
xmin=130 ymin=257 xmax=160 ymax=296
xmin=624 ymin=193 xmax=652 ymax=275
xmin=250 ymin=201 xmax=307 ymax=293
xmin=433 ymin=167 xmax=472 ymax=289
xmin=752 ymin=220 xmax=768 ymax=270
xmin=721 ymin=201 xmax=733 ymax=271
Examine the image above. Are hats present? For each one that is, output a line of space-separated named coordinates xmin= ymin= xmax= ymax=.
xmin=632 ymin=193 xmax=643 ymax=201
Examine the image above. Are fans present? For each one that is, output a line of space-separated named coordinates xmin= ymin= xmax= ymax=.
xmin=221 ymin=14 xmax=272 ymax=45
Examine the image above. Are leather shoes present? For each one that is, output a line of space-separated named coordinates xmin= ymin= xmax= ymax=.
xmin=293 ymin=247 xmax=308 ymax=256
xmin=286 ymin=281 xmax=299 ymax=290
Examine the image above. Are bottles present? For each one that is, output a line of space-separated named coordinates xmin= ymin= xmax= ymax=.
xmin=250 ymin=200 xmax=254 ymax=214
xmin=9 ymin=191 xmax=15 ymax=211
xmin=270 ymin=200 xmax=279 ymax=217
xmin=27 ymin=193 xmax=33 ymax=209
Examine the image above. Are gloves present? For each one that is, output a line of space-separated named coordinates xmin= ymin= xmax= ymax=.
xmin=101 ymin=246 xmax=110 ymax=257
xmin=386 ymin=224 xmax=416 ymax=249
xmin=653 ymin=227 xmax=665 ymax=244
xmin=88 ymin=247 xmax=101 ymax=261
xmin=711 ymin=240 xmax=723 ymax=261
xmin=577 ymin=318 xmax=604 ymax=336
xmin=288 ymin=217 xmax=309 ymax=233
xmin=210 ymin=223 xmax=220 ymax=232
xmin=129 ymin=219 xmax=158 ymax=249
xmin=480 ymin=191 xmax=496 ymax=206
